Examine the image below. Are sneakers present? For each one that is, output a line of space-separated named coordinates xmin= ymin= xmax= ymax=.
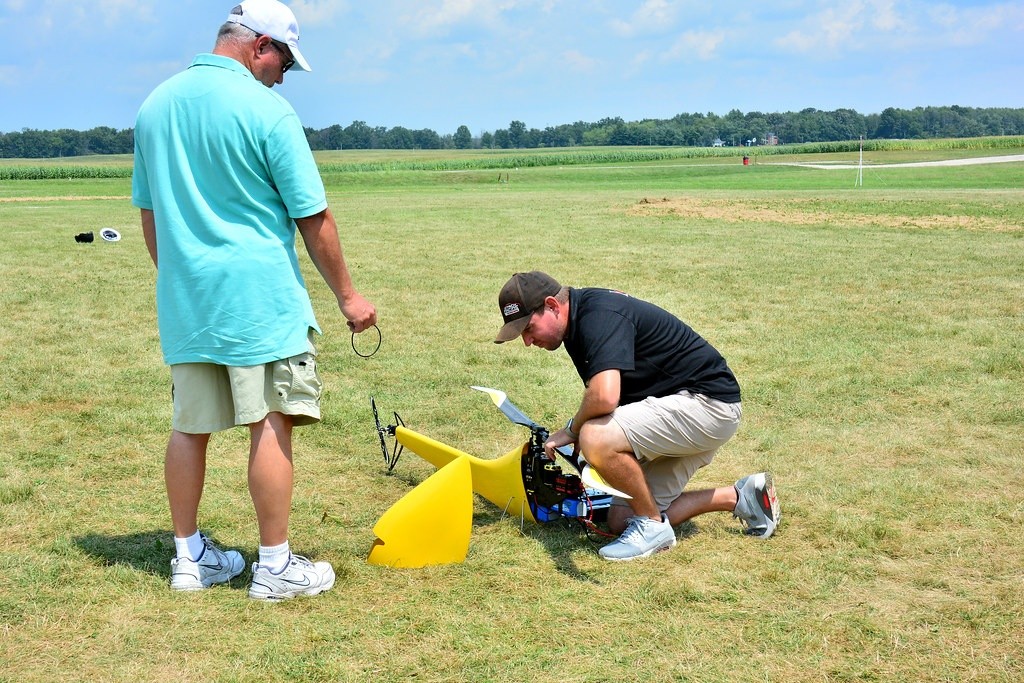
xmin=249 ymin=550 xmax=335 ymax=604
xmin=171 ymin=534 xmax=246 ymax=589
xmin=598 ymin=512 xmax=677 ymax=563
xmin=729 ymin=473 xmax=780 ymax=540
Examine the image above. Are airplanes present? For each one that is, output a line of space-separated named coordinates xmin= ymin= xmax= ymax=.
xmin=365 ymin=386 xmax=634 ymax=571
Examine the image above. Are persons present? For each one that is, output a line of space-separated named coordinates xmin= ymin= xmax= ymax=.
xmin=131 ymin=0 xmax=378 ymax=601
xmin=493 ymin=271 xmax=781 ymax=562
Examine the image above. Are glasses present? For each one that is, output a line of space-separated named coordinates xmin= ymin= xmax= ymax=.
xmin=255 ymin=36 xmax=296 ymax=73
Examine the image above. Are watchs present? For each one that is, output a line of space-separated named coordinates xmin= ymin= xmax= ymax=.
xmin=564 ymin=417 xmax=579 ymax=440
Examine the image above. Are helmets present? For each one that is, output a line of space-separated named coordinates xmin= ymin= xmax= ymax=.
xmin=74 ymin=231 xmax=94 ymax=243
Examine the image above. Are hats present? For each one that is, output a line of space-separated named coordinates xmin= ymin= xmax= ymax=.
xmin=494 ymin=271 xmax=562 ymax=344
xmin=226 ymin=0 xmax=312 ymax=73
xmin=100 ymin=228 xmax=121 ymax=241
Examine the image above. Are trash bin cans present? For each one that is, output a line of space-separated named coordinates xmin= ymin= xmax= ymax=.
xmin=743 ymin=157 xmax=748 ymax=165
